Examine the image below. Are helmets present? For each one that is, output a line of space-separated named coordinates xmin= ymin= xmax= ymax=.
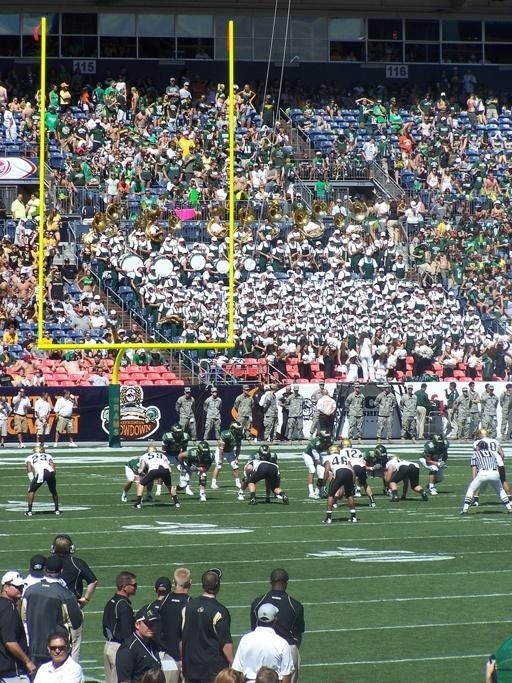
xmin=33 ymin=444 xmax=45 ymax=454
xmin=170 ymin=421 xmax=388 ymax=456
xmin=431 ymin=434 xmax=444 ymax=447
xmin=479 ymin=427 xmax=489 ymax=437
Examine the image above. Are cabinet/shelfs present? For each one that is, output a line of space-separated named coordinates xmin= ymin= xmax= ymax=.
xmin=424 ymin=409 xmax=446 ymax=440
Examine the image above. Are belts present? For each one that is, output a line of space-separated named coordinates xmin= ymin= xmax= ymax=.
xmin=105 ymin=638 xmax=122 ymax=643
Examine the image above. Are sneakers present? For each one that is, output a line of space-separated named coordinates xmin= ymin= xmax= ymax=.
xmin=348 ymin=516 xmax=358 ymax=523
xmin=54 ymin=510 xmax=61 ymax=515
xmin=321 ymin=518 xmax=332 ymax=524
xmin=24 ymin=511 xmax=32 ymax=517
xmin=121 ymin=481 xmax=512 ymax=515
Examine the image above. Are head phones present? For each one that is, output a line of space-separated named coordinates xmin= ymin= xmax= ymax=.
xmin=50 ymin=535 xmax=75 ymax=555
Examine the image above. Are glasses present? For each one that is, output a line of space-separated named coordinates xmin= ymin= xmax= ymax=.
xmin=5 ymin=584 xmax=24 ymax=591
xmin=123 ymin=583 xmax=138 ymax=589
xmin=48 ymin=645 xmax=68 ymax=651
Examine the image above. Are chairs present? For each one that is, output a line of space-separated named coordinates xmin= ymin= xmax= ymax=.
xmin=401 ymin=170 xmax=415 ymax=189
xmin=140 ymin=308 xmax=180 ymax=343
xmin=127 ymin=193 xmax=142 ymax=213
xmin=1 ymin=110 xmax=65 ymax=171
xmin=427 ymin=362 xmax=509 ymax=382
xmin=7 ymin=345 xmax=23 ymax=360
xmin=190 ymin=350 xmax=214 ymax=360
xmin=19 ymin=322 xmax=105 ymax=344
xmin=150 ymin=181 xmax=169 ymax=197
xmin=66 ymin=283 xmax=82 ymax=302
xmin=458 ymin=111 xmax=512 ymax=162
xmin=288 ymin=109 xmax=422 ymax=158
xmin=69 ymin=105 xmax=85 ymax=119
xmin=158 ymin=220 xmax=214 ymax=244
xmin=396 ymin=357 xmax=414 ymax=382
xmin=103 ymin=279 xmax=135 ymax=308
xmin=6 ymin=357 xmax=186 ymax=385
xmin=224 ymin=358 xmax=346 ymax=383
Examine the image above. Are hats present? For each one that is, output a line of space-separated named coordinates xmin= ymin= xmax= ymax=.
xmin=270 ymin=381 xmax=300 ymax=390
xmin=30 ymin=554 xmax=47 ymax=571
xmin=1 ymin=570 xmax=28 ymax=588
xmin=319 ymin=380 xmax=325 ymax=385
xmin=257 ymin=603 xmax=280 ymax=622
xmin=154 ymin=576 xmax=171 ymax=593
xmin=354 ymin=383 xmax=360 ymax=388
xmin=135 ymin=607 xmax=159 ymax=623
xmin=487 ymin=385 xmax=495 ymax=390
xmin=201 ymin=567 xmax=222 ymax=590
xmin=408 ymin=385 xmax=413 ymax=389
xmin=93 ymin=224 xmax=484 ymax=341
xmin=462 ymin=387 xmax=468 ymax=392
xmin=209 ymin=388 xmax=219 ymax=393
xmin=242 ymin=385 xmax=250 ymax=390
xmin=184 ymin=387 xmax=191 ymax=393
xmin=45 ymin=555 xmax=63 ymax=573
xmin=384 ymin=383 xmax=391 ymax=388
